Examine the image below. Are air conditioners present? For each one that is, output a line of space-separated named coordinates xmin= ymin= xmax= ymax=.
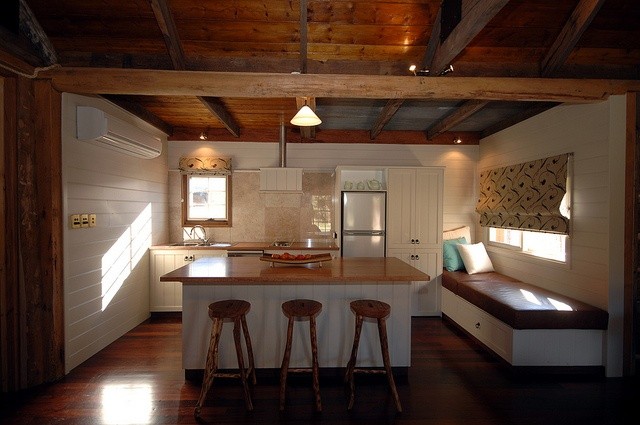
xmin=75 ymin=105 xmax=163 ymax=160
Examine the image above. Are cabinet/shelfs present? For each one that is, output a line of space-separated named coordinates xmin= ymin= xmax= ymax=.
xmin=148 ymin=250 xmax=230 ymax=313
xmin=266 ymin=250 xmax=338 ymax=260
xmin=442 ymin=270 xmax=609 ymax=367
xmin=385 ymin=165 xmax=448 ymax=249
xmin=385 ymin=247 xmax=444 ymax=318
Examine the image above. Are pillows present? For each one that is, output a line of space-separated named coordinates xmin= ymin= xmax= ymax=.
xmin=443 ymin=226 xmax=472 ymax=244
xmin=456 ymin=242 xmax=496 ymax=276
xmin=443 ymin=236 xmax=468 ymax=273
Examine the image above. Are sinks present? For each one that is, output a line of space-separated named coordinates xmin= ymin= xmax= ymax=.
xmin=171 ymin=243 xmax=197 ymax=246
xmin=199 ymin=244 xmax=213 ymax=246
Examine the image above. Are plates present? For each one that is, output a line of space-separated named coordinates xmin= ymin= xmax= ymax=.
xmin=261 ymin=252 xmax=331 ymax=268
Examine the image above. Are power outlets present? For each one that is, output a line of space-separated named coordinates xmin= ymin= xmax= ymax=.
xmin=70 ymin=213 xmax=96 ymax=229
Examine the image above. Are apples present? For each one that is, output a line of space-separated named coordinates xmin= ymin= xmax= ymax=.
xmin=284 ymin=253 xmax=289 ymax=256
xmin=305 ymin=254 xmax=311 ymax=259
xmin=271 ymin=254 xmax=280 ymax=259
xmin=280 ymin=255 xmax=288 ymax=260
xmin=289 ymin=255 xmax=295 ymax=260
xmin=297 ymin=255 xmax=305 ymax=260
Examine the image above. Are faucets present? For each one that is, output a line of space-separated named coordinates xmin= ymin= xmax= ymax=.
xmin=190 ymin=225 xmax=207 ymax=243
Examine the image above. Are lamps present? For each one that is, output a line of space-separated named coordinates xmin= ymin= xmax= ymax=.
xmin=407 ymin=63 xmax=455 ymax=78
xmin=198 ymin=126 xmax=210 ymax=141
xmin=289 ymin=97 xmax=323 ymax=127
xmin=453 ymin=134 xmax=462 ymax=144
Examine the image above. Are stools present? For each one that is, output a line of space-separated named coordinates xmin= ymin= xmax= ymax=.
xmin=279 ymin=298 xmax=324 ymax=415
xmin=193 ymin=299 xmax=259 ymax=420
xmin=341 ymin=300 xmax=403 ymax=415
